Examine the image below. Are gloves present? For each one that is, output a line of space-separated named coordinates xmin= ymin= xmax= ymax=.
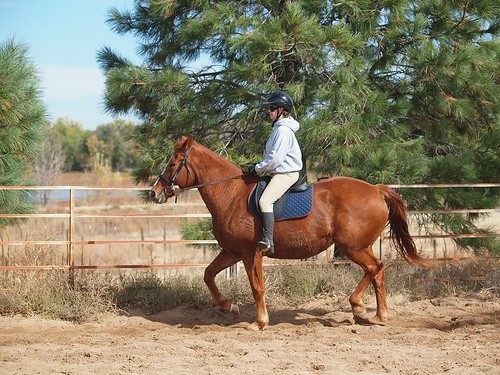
xmin=248 ymin=166 xmax=256 ymax=175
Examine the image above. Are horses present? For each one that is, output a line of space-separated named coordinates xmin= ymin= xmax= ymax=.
xmin=149 ymin=134 xmax=432 ymax=332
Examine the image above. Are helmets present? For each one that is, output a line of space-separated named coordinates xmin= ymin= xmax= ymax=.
xmin=266 ymin=91 xmax=292 ymax=112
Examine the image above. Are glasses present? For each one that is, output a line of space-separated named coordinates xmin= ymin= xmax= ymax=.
xmin=269 ymin=106 xmax=279 ymax=111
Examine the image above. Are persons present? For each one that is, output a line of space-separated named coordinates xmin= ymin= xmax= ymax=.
xmin=247 ymin=91 xmax=303 ymax=257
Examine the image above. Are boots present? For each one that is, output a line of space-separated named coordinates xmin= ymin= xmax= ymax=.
xmin=257 ymin=212 xmax=274 ymax=256
